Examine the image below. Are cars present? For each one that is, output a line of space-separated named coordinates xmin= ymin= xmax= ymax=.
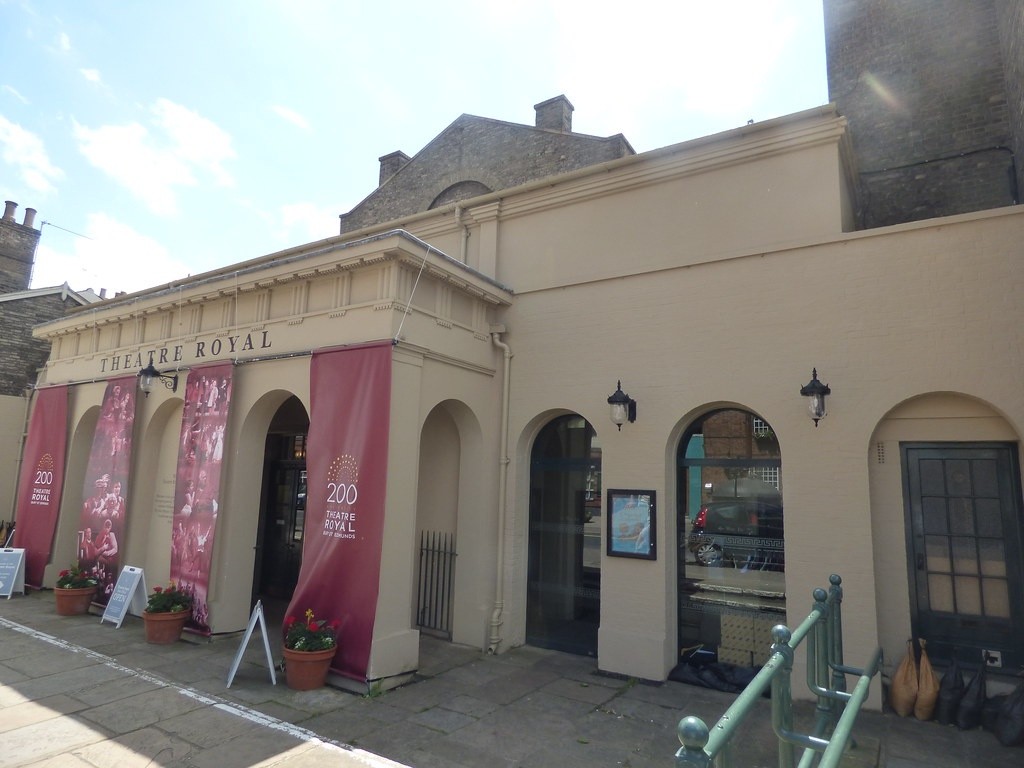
xmin=688 ymin=498 xmax=785 ymax=567
xmin=297 ymin=491 xmax=306 ymax=508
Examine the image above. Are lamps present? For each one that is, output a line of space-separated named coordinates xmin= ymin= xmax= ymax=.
xmin=799 ymin=367 xmax=831 ymax=428
xmin=136 ymin=356 xmax=179 ymax=399
xmin=607 ymin=379 xmax=637 ymax=431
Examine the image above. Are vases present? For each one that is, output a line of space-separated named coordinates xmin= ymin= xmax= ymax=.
xmin=142 ymin=605 xmax=192 ymax=646
xmin=53 ymin=585 xmax=98 ymax=617
xmin=280 ymin=642 xmax=337 ymax=690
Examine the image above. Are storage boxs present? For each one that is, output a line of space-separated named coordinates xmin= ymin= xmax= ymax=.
xmin=716 ymin=610 xmax=786 ymax=667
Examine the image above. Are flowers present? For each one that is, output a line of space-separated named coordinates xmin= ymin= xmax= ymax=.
xmin=56 ymin=565 xmax=98 ymax=589
xmin=283 ymin=608 xmax=343 ymax=652
xmin=147 ymin=578 xmax=195 ymax=613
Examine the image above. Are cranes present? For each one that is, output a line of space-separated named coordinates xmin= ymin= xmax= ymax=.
xmin=28 ymin=221 xmax=90 ymax=289
